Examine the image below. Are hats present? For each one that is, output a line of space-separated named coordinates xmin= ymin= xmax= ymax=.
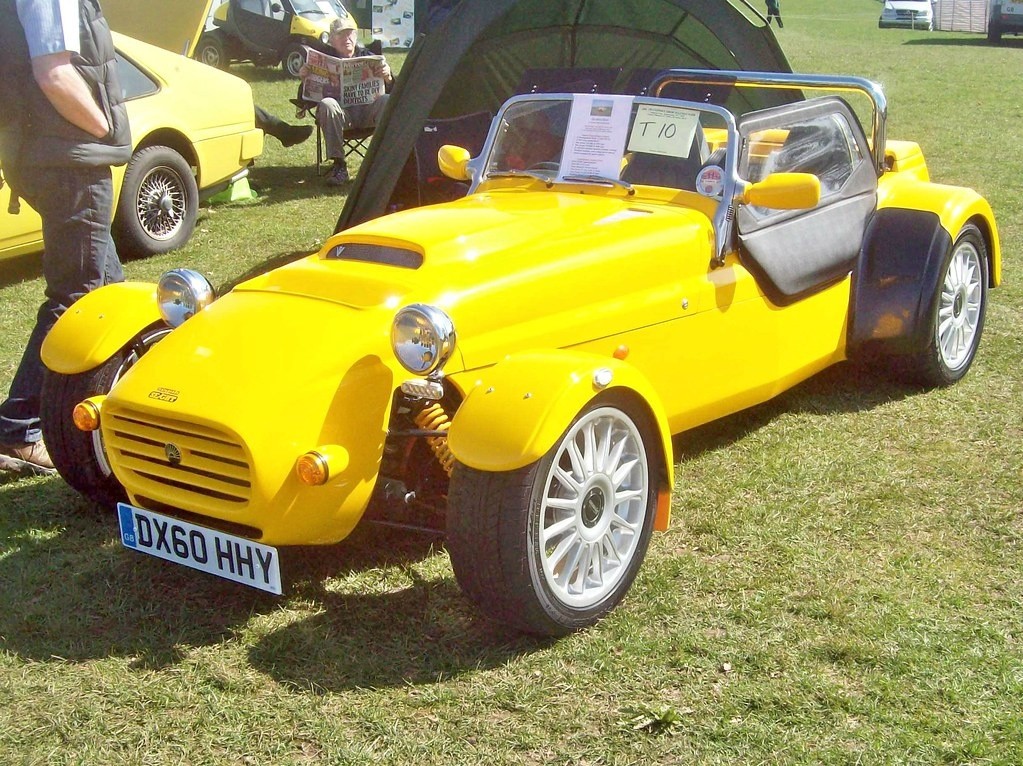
xmin=329 ymin=17 xmax=357 ymax=36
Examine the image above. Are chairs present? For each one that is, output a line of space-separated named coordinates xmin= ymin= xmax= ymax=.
xmin=413 ymin=110 xmax=494 ymax=206
xmin=616 ymin=117 xmax=712 ymax=193
xmin=773 ymin=122 xmax=850 ymax=190
xmin=308 ymin=99 xmax=376 ymax=178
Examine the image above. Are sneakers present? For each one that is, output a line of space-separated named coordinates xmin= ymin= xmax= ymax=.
xmin=0 ymin=438 xmax=57 ymax=474
xmin=323 ymin=162 xmax=349 ymax=185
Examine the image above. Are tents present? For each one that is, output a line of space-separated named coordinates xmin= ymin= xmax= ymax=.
xmin=333 ymin=0 xmax=806 ymax=236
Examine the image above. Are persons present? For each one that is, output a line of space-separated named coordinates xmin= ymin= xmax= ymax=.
xmin=254 ymin=104 xmax=314 ymax=147
xmin=296 ymin=18 xmax=397 ymax=186
xmin=765 ymin=0 xmax=783 ymax=28
xmin=0 ymin=0 xmax=132 ymax=477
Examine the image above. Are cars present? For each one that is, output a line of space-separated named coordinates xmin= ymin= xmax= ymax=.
xmin=1 ymin=0 xmax=265 ymax=261
xmin=879 ymin=0 xmax=934 ymax=31
xmin=193 ymin=0 xmax=358 ymax=79
xmin=41 ymin=67 xmax=1000 ymax=639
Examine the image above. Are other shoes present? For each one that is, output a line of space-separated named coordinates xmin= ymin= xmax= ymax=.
xmin=281 ymin=126 xmax=313 ymax=147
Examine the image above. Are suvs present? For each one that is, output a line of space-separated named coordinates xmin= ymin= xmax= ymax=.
xmin=988 ymin=0 xmax=1023 ymax=42
